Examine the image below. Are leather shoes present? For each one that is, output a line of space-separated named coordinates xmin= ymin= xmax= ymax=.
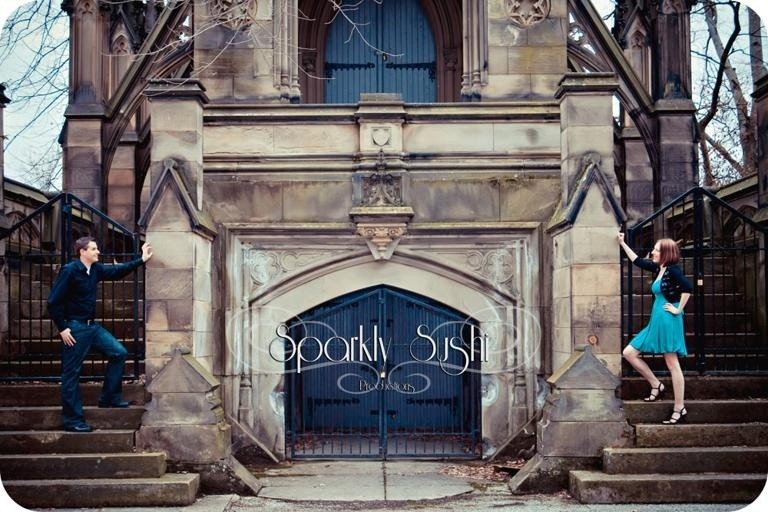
xmin=98 ymin=399 xmax=128 ymax=408
xmin=67 ymin=423 xmax=94 ymax=432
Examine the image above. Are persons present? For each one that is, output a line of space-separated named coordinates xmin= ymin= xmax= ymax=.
xmin=614 ymin=227 xmax=695 ymax=424
xmin=45 ymin=235 xmax=153 ymax=431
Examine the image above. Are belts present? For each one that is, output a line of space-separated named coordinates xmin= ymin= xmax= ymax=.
xmin=66 ymin=318 xmax=93 ymax=327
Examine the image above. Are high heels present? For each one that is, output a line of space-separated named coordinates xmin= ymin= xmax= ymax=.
xmin=663 ymin=408 xmax=687 ymax=424
xmin=644 ymin=383 xmax=664 ymax=402
